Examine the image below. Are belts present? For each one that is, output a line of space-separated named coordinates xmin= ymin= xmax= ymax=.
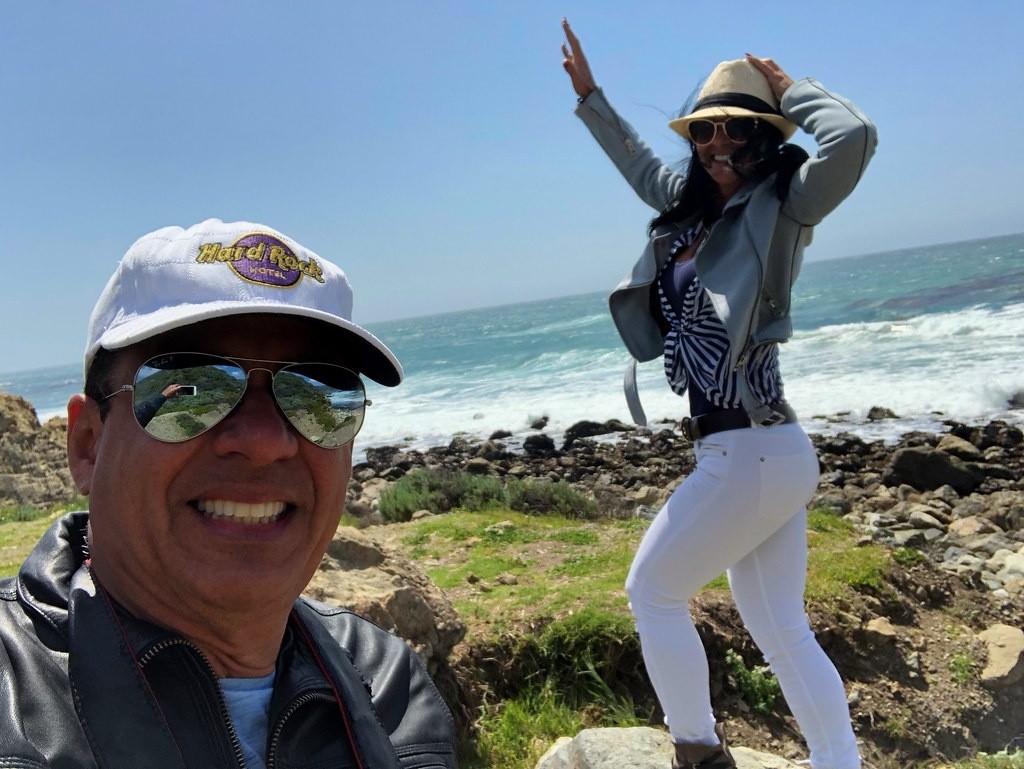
xmin=684 ymin=403 xmax=798 ymax=440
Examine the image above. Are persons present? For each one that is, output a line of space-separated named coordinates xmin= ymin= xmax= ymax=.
xmin=560 ymin=14 xmax=879 ymax=768
xmin=0 ymin=217 xmax=470 ymax=769
xmin=134 ymin=384 xmax=181 ymax=430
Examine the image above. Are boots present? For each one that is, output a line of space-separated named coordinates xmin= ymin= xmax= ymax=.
xmin=671 ymin=723 xmax=738 ymax=769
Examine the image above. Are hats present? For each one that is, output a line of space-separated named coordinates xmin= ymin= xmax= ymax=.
xmin=668 ymin=59 xmax=797 ymax=142
xmin=84 ymin=218 xmax=404 ymax=392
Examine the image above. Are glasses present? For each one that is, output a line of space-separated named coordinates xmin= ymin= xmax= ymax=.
xmin=687 ymin=116 xmax=758 ymax=146
xmin=103 ymin=351 xmax=372 ymax=449
